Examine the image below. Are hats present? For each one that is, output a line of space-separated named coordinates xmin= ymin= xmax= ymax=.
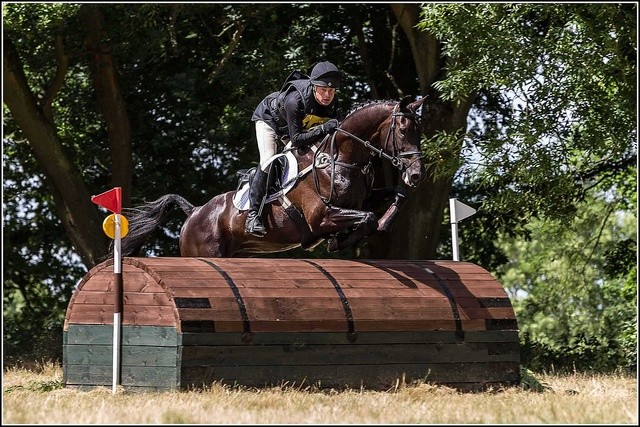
xmin=308 ymin=61 xmax=341 ymax=105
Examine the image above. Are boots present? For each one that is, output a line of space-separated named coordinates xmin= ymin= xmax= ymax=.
xmin=244 ymin=164 xmax=271 ymax=237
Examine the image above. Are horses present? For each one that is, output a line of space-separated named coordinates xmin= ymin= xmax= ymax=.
xmin=95 ymin=94 xmax=431 ymax=265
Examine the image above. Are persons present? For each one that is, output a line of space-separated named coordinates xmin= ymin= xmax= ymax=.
xmin=245 ymin=61 xmax=341 ymax=234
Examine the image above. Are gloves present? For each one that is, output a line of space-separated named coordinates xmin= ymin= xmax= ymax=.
xmin=322 ymin=119 xmax=339 ymax=136
